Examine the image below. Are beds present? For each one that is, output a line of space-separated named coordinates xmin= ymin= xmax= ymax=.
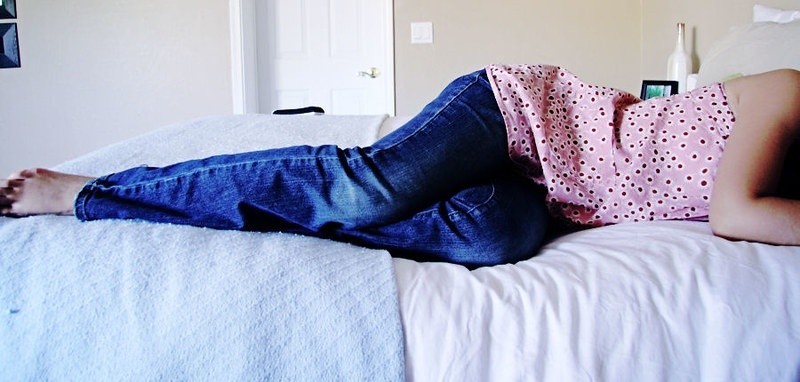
xmin=0 ymin=113 xmax=800 ymax=382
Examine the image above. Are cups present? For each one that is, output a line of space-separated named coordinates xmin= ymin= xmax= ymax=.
xmin=687 ymin=73 xmax=697 ymax=92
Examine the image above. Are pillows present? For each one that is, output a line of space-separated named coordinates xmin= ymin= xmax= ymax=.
xmin=696 ymin=19 xmax=800 ymax=88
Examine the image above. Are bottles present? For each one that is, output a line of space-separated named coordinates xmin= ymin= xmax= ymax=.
xmin=667 ymin=22 xmax=692 ymax=94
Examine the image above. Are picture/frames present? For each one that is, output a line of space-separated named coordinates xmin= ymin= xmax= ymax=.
xmin=640 ymin=80 xmax=678 ymax=101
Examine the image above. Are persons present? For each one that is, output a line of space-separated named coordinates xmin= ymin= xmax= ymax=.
xmin=0 ymin=64 xmax=800 ymax=271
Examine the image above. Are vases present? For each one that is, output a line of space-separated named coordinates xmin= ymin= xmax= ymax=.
xmin=667 ymin=23 xmax=692 ymax=94
